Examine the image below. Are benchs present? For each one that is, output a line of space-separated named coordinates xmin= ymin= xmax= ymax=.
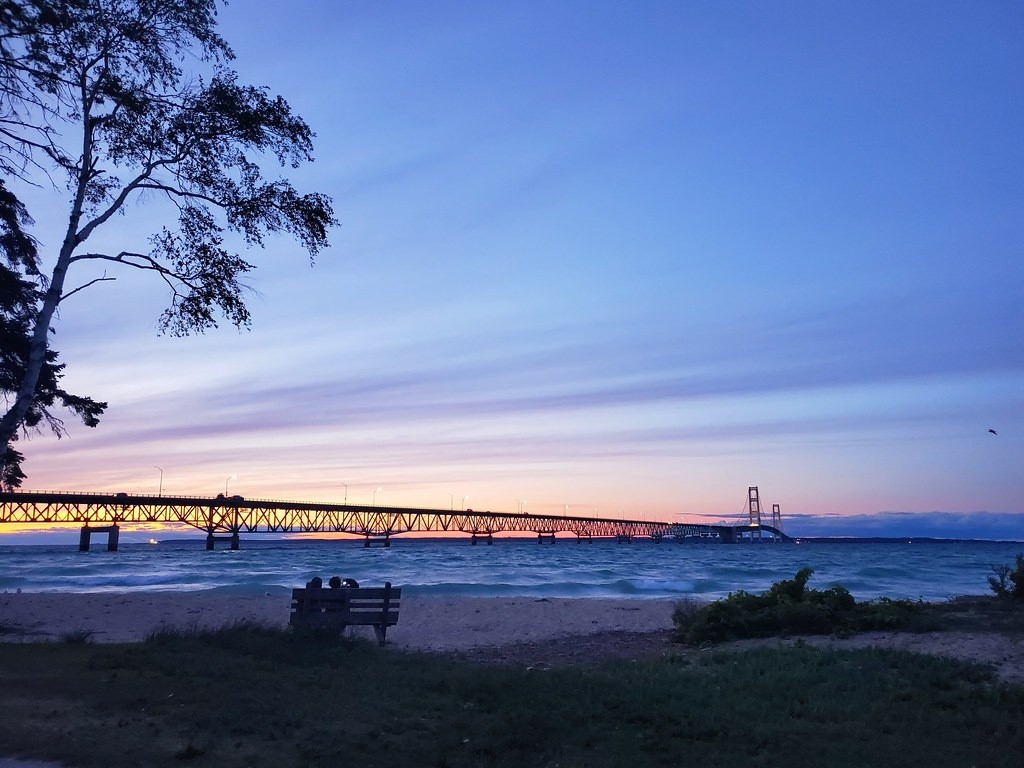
xmin=287 ymin=582 xmax=402 ymax=647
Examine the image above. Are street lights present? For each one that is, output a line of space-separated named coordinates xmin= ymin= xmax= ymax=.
xmin=226 ymin=475 xmax=235 ymax=497
xmin=373 ymin=488 xmax=381 ymax=506
xmin=154 ymin=465 xmax=163 ymax=496
xmin=341 ymin=482 xmax=347 ymax=505
xmin=461 ymin=496 xmax=469 ymax=511
xmin=562 ymin=505 xmax=569 ymax=516
xmin=592 ymin=507 xmax=663 ymax=522
xmin=516 ymin=498 xmax=526 ymax=514
xmin=449 ymin=492 xmax=453 ymax=510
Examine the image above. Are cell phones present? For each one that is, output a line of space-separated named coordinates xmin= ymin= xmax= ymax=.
xmin=343 ymin=582 xmax=346 ymax=586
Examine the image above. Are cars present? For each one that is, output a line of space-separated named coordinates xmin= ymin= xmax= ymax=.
xmin=228 ymin=495 xmax=244 ymax=501
xmin=467 ymin=509 xmax=473 ymax=512
xmin=524 ymin=512 xmax=528 ymax=514
xmin=114 ymin=493 xmax=127 ymax=497
xmin=486 ymin=511 xmax=490 ymax=513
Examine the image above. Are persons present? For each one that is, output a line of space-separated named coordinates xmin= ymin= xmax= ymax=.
xmin=325 ymin=576 xmax=351 ymax=616
xmin=296 ymin=577 xmax=322 ymax=613
